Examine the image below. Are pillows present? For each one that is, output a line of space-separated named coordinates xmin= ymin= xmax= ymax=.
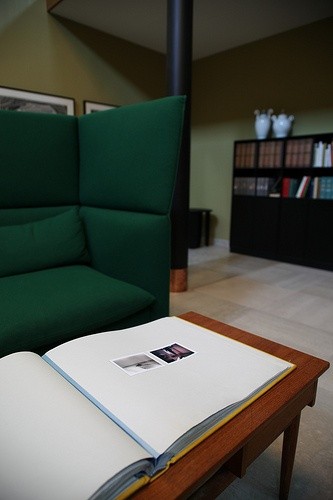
xmin=0 ymin=207 xmax=90 ymax=276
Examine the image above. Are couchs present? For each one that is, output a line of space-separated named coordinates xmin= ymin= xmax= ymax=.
xmin=0 ymin=95 xmax=187 ymax=359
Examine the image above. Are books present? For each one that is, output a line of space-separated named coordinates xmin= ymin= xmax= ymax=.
xmin=258 ymin=141 xmax=282 ymax=168
xmin=313 ymin=141 xmax=333 ymax=167
xmin=285 ymin=139 xmax=312 ymax=168
xmin=234 ymin=177 xmax=255 ymax=195
xmin=312 ymin=176 xmax=333 ymax=199
xmin=0 ymin=316 xmax=296 ymax=500
xmin=235 ymin=143 xmax=255 ymax=168
xmin=283 ymin=176 xmax=311 ymax=198
xmin=257 ymin=177 xmax=281 ymax=197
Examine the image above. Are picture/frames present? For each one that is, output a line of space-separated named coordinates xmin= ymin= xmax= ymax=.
xmin=0 ymin=86 xmax=76 ymax=116
xmin=82 ymin=100 xmax=120 ymax=115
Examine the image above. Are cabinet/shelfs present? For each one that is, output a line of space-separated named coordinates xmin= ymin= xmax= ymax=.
xmin=229 ymin=131 xmax=333 ymax=273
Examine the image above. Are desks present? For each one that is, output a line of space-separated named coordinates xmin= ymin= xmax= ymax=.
xmin=126 ymin=311 xmax=330 ymax=500
